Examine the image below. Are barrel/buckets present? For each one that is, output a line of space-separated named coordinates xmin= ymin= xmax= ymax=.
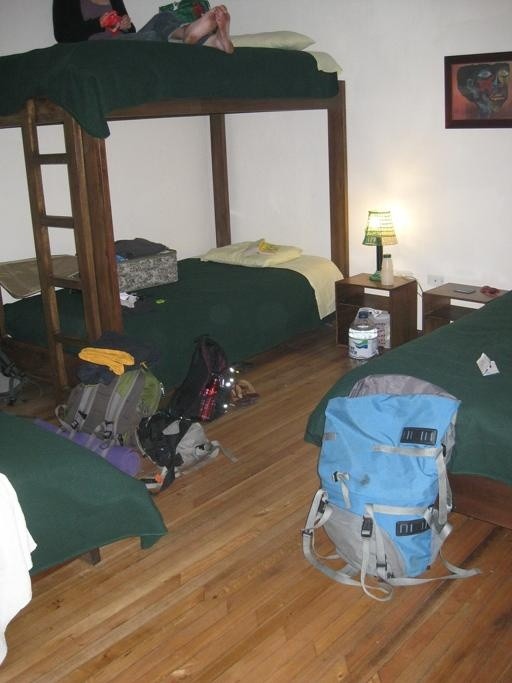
xmin=348 ymin=312 xmax=378 ymax=369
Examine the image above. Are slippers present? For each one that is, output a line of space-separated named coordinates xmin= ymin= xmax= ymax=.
xmin=238 ymin=379 xmax=259 ymax=399
xmin=231 ymin=385 xmax=248 ymax=406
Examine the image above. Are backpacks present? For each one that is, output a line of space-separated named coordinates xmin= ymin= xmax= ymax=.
xmin=303 ymin=374 xmax=480 ymax=601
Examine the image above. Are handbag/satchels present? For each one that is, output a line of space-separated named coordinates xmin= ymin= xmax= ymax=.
xmin=27 ymin=336 xmax=232 ymax=489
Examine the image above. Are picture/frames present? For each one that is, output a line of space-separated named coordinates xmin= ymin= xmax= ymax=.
xmin=443 ymin=52 xmax=512 ymax=132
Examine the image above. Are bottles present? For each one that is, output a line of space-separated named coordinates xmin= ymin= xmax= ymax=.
xmin=380 ymin=252 xmax=394 ymax=287
xmin=347 ymin=310 xmax=380 ymax=370
xmin=216 ymin=366 xmax=237 ymax=416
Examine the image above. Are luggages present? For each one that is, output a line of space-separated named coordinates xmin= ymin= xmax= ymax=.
xmin=0 ymin=238 xmax=178 ymax=299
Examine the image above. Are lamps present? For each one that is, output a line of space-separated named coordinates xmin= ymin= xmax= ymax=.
xmin=361 ymin=210 xmax=399 ymax=283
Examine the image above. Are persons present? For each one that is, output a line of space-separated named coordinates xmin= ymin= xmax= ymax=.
xmin=53 ymin=0 xmax=234 ymax=54
xmin=456 ymin=62 xmax=509 ymax=118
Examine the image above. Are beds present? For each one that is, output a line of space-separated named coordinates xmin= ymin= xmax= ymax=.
xmin=0 ymin=38 xmax=344 ymax=408
xmin=302 ymin=292 xmax=512 ymax=531
xmin=0 ymin=413 xmax=169 ymax=585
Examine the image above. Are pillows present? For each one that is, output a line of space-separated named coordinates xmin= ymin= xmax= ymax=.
xmin=222 ymin=31 xmax=316 ymax=50
xmin=197 ymin=238 xmax=305 ymax=270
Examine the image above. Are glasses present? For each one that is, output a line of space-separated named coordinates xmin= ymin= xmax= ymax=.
xmin=480 ymin=286 xmax=498 ymax=297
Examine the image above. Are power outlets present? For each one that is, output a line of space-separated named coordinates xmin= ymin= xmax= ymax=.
xmin=426 ymin=273 xmax=446 ymax=291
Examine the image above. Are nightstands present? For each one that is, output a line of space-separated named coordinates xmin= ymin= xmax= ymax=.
xmin=422 ymin=282 xmax=507 ymax=335
xmin=334 ymin=272 xmax=419 ymax=349
